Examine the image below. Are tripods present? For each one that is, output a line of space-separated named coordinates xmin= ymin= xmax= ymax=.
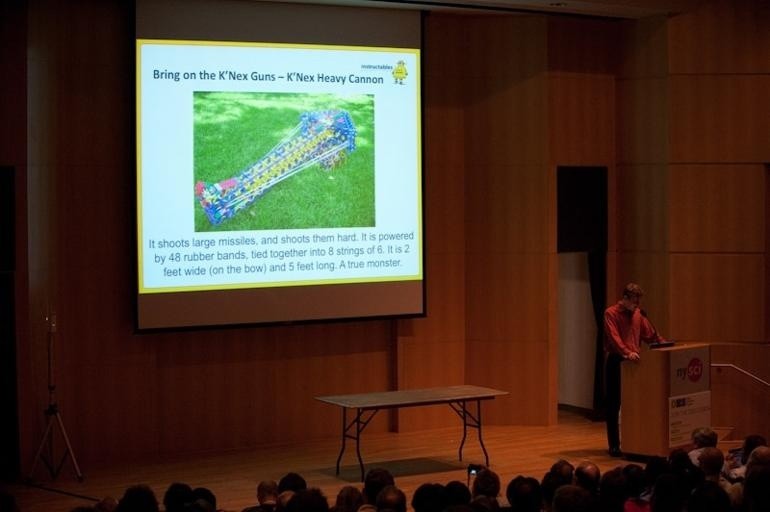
xmin=25 ymin=313 xmax=86 ymax=486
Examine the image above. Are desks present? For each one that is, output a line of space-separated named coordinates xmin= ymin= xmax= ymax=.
xmin=313 ymin=383 xmax=511 ymax=482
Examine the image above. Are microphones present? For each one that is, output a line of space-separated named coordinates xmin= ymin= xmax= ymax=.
xmin=639 ymin=309 xmax=674 ymax=349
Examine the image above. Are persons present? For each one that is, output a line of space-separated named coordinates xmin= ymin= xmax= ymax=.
xmin=410 ymin=422 xmax=770 ymax=512
xmin=601 ymin=282 xmax=666 ymax=458
xmin=244 ymin=468 xmax=407 ymax=512
xmin=96 ymin=483 xmax=217 ymax=512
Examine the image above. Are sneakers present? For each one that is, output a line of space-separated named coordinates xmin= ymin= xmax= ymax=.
xmin=605 ymin=447 xmax=624 ymax=458
